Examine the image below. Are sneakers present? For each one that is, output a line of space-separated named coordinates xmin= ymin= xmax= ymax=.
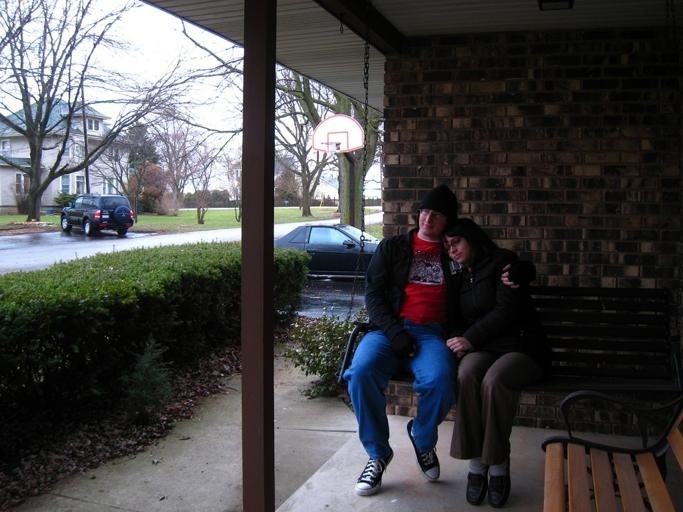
xmin=488 ymin=457 xmax=511 ymax=508
xmin=466 ymin=465 xmax=488 ymax=506
xmin=407 ymin=419 xmax=440 ymax=481
xmin=354 ymin=450 xmax=394 ymax=494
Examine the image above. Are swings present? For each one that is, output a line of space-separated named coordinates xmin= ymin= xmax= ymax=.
xmin=334 ymin=23 xmax=682 ymax=396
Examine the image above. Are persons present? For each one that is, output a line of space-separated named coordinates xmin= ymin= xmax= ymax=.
xmin=341 ymin=184 xmax=536 ymax=496
xmin=442 ymin=217 xmax=554 ymax=509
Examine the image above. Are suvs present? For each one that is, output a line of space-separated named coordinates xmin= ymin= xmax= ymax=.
xmin=60 ymin=193 xmax=134 ymax=235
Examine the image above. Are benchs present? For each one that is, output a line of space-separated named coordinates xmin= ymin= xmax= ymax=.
xmin=537 ymin=390 xmax=683 ymax=509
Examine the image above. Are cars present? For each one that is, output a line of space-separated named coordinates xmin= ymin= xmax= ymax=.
xmin=274 ymin=224 xmax=383 ymax=275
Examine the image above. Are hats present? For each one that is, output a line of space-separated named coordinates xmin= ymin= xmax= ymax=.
xmin=416 ymin=183 xmax=457 ymax=228
